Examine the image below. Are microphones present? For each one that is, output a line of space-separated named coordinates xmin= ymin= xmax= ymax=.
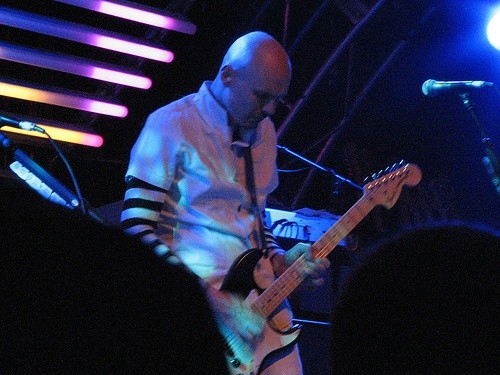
xmin=422 ymin=78 xmax=496 ymax=98
xmin=0 ymin=115 xmax=45 ymax=134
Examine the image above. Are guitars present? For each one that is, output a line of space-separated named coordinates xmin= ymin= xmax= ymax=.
xmin=210 ymin=159 xmax=422 ymax=375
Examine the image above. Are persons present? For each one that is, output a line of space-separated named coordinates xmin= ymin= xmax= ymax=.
xmin=117 ymin=30 xmax=331 ymax=375
